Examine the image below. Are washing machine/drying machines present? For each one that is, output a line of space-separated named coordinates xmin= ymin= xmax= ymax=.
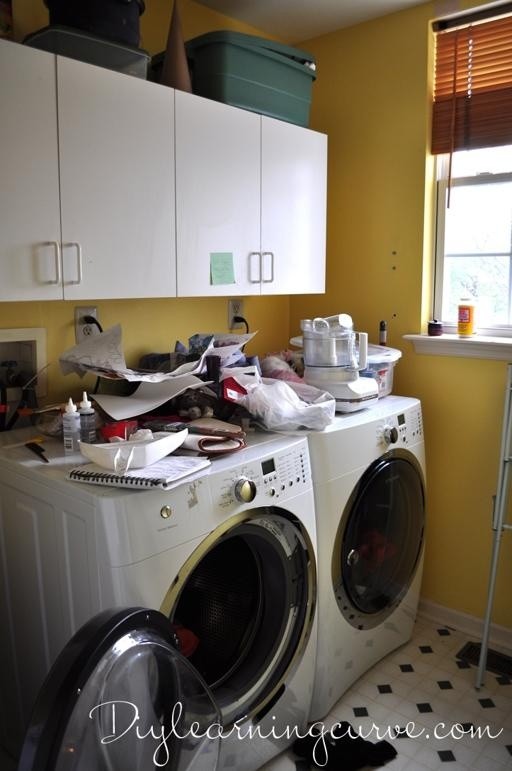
xmin=1 ymin=425 xmax=319 ymax=770
xmin=308 ymin=395 xmax=428 ymax=723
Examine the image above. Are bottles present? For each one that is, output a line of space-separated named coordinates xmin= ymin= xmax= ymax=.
xmin=78 ymin=391 xmax=97 ymax=445
xmin=457 ymin=296 xmax=475 ymax=339
xmin=62 ymin=397 xmax=82 ymax=453
xmin=427 ymin=319 xmax=444 ymax=336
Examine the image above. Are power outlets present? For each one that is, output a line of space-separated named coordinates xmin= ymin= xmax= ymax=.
xmin=229 ymin=300 xmax=245 ymax=330
xmin=74 ymin=307 xmax=98 ymax=343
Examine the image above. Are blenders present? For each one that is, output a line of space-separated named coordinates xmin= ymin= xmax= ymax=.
xmin=299 ymin=313 xmax=379 ymax=414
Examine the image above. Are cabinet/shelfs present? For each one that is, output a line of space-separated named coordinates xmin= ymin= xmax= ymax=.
xmin=0 ymin=38 xmax=328 ymax=301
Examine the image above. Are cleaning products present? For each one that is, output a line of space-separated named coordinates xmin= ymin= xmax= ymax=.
xmin=63 ymin=400 xmax=80 ymax=450
xmin=76 ymin=392 xmax=97 ymax=440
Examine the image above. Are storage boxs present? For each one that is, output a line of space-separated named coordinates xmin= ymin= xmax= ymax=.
xmin=289 ymin=334 xmax=402 ymax=400
xmin=148 ymin=29 xmax=317 ymax=128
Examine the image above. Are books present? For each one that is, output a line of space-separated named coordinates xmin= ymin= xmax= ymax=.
xmin=65 ymin=455 xmax=212 ymax=491
